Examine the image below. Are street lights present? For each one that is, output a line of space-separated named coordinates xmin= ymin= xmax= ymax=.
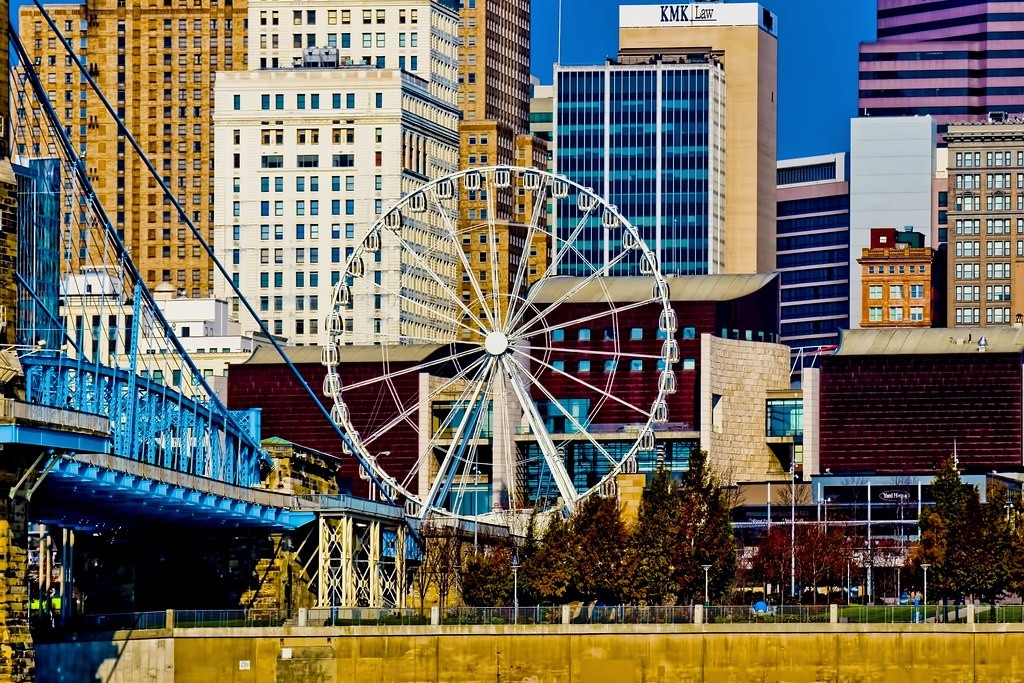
xmin=509 ymin=565 xmax=522 ymax=624
xmin=700 ymin=564 xmax=712 ymax=623
xmin=919 ymin=563 xmax=931 ymax=624
